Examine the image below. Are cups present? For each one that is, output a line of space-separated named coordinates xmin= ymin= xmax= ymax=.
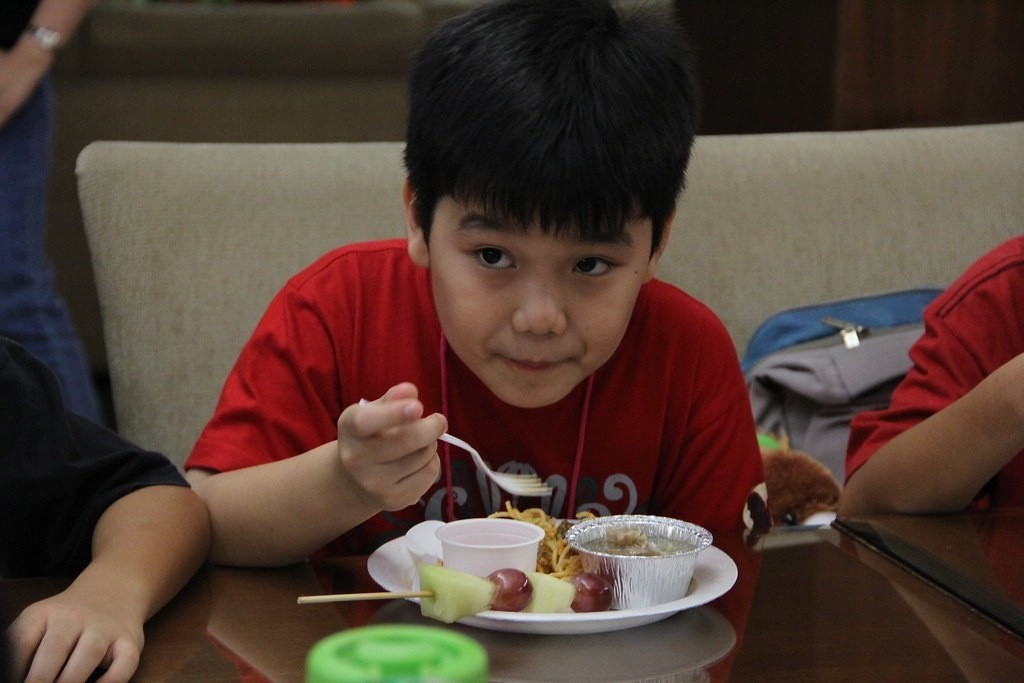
xmin=565 ymin=514 xmax=713 ymax=611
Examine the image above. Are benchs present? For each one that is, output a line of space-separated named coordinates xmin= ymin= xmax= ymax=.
xmin=75 ymin=124 xmax=1023 ymax=476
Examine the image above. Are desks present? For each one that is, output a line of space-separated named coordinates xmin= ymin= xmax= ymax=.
xmin=132 ymin=499 xmax=1024 ymax=683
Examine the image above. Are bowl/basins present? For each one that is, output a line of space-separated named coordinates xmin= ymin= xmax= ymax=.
xmin=436 ymin=518 xmax=546 ymax=579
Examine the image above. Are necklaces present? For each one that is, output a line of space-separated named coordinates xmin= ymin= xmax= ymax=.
xmin=440 ymin=330 xmax=594 ymax=521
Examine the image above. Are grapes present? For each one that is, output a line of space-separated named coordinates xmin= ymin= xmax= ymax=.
xmin=486 ymin=568 xmax=533 ymax=611
xmin=568 ymin=572 xmax=614 ymax=613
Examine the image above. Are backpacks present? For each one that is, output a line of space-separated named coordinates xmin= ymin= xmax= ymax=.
xmin=740 ymin=289 xmax=946 ymax=491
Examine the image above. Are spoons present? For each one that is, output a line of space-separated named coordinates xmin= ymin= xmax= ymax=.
xmin=405 ymin=520 xmax=446 ymax=596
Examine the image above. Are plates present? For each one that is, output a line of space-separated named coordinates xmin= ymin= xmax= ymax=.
xmin=366 ymin=521 xmax=738 ymax=634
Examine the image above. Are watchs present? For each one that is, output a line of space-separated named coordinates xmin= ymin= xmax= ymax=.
xmin=24 ymin=22 xmax=69 ymax=60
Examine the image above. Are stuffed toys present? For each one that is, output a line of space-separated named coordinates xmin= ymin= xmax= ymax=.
xmin=759 ymin=448 xmax=840 ymax=534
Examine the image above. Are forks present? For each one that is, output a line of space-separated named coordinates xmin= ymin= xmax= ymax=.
xmin=358 ymin=398 xmax=554 ymax=497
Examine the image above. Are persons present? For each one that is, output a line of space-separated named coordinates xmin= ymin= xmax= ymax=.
xmin=184 ymin=0 xmax=770 ymax=573
xmin=0 ymin=0 xmax=104 ymax=423
xmin=1 ymin=345 xmax=212 ymax=683
xmin=840 ymin=234 xmax=1024 ymax=519
